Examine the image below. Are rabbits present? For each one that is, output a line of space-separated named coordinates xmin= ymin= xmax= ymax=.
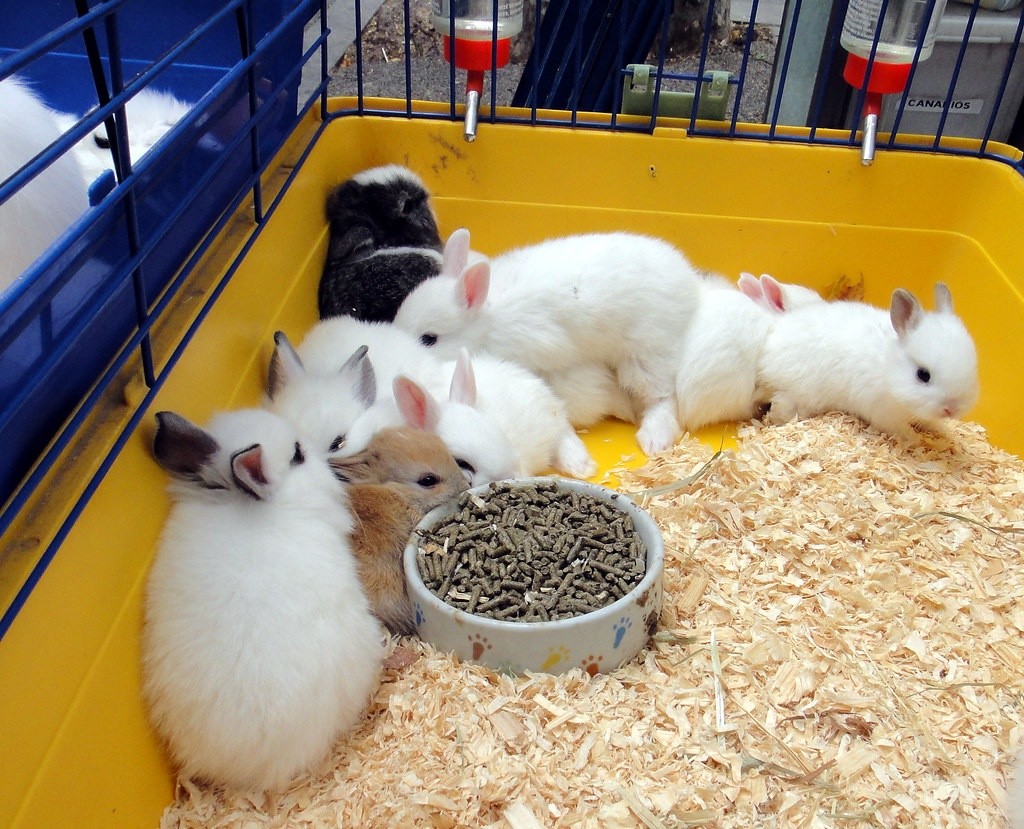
xmin=0 ymin=76 xmax=204 ymax=297
xmin=142 ymin=163 xmax=980 ymax=802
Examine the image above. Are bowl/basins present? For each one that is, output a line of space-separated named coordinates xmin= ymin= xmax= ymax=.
xmin=402 ymin=474 xmax=664 ymax=682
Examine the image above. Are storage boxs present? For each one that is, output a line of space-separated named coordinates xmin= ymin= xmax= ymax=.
xmin=861 ymin=3 xmax=1024 ymax=149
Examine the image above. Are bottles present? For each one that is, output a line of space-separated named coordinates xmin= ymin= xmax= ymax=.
xmin=428 ymin=0 xmax=523 ymax=70
xmin=840 ymin=0 xmax=944 ymax=95
